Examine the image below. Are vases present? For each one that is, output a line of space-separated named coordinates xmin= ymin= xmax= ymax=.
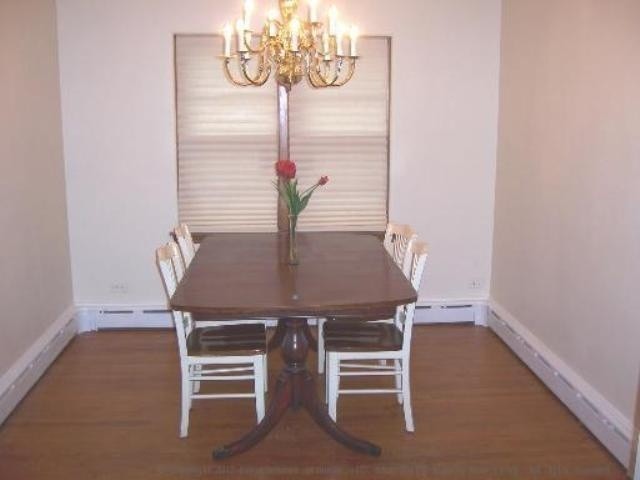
xmin=286 ymin=216 xmax=300 ymax=265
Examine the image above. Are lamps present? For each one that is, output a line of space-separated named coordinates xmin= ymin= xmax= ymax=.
xmin=217 ymin=0 xmax=359 ymax=90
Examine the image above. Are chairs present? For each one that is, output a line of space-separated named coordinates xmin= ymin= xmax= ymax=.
xmin=156 ymin=243 xmax=267 ymax=438
xmin=324 ymin=241 xmax=428 ymax=432
xmin=319 ymin=223 xmax=417 ymax=375
xmin=174 ymin=223 xmax=278 ymax=393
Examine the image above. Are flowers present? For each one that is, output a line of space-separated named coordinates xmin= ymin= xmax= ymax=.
xmin=272 ymin=160 xmax=328 ymax=259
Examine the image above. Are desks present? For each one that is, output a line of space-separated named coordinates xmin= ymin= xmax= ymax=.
xmin=170 ymin=234 xmax=418 ymax=459
